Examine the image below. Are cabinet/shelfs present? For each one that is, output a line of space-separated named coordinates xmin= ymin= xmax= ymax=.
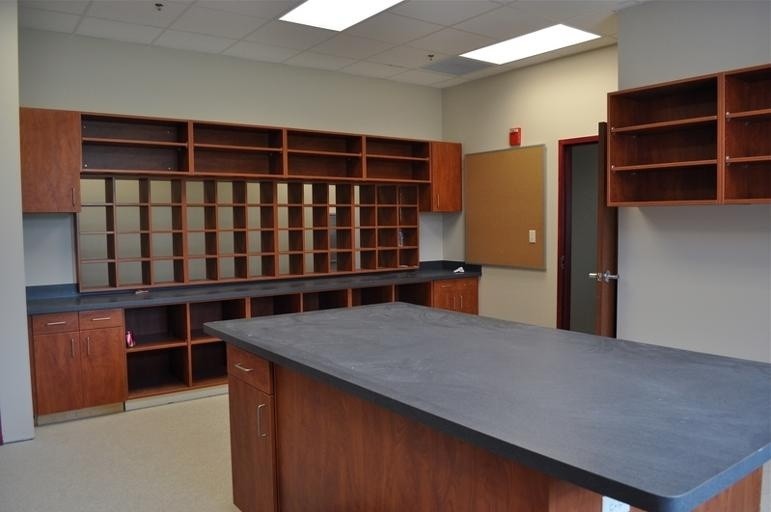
xmin=606 ymin=62 xmax=771 ymax=208
xmin=225 ymin=343 xmax=279 ymax=511
xmin=19 ymin=107 xmax=481 ymax=426
